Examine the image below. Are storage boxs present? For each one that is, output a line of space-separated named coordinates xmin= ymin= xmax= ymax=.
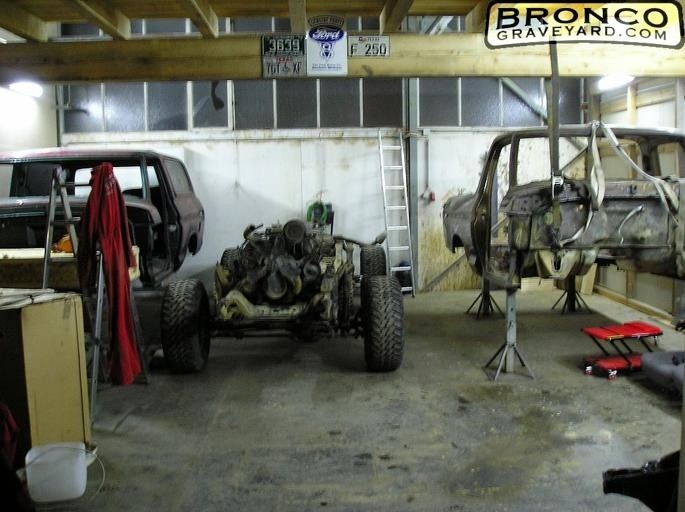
xmin=24 ymin=441 xmax=87 ymax=503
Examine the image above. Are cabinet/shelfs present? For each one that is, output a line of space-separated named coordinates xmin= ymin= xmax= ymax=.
xmin=0 ymin=246 xmax=141 ymax=293
xmin=0 ymin=288 xmax=93 ymax=461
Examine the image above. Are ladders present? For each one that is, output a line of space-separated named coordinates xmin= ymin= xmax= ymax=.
xmin=377 ymin=128 xmax=415 ymax=298
xmin=43 ymin=165 xmax=152 ymax=430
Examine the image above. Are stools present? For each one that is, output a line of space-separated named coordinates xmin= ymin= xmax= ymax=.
xmin=581 ymin=320 xmax=663 ymax=377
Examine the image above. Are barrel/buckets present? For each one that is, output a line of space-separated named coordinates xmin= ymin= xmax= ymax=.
xmin=17 ymin=440 xmax=99 ymax=503
